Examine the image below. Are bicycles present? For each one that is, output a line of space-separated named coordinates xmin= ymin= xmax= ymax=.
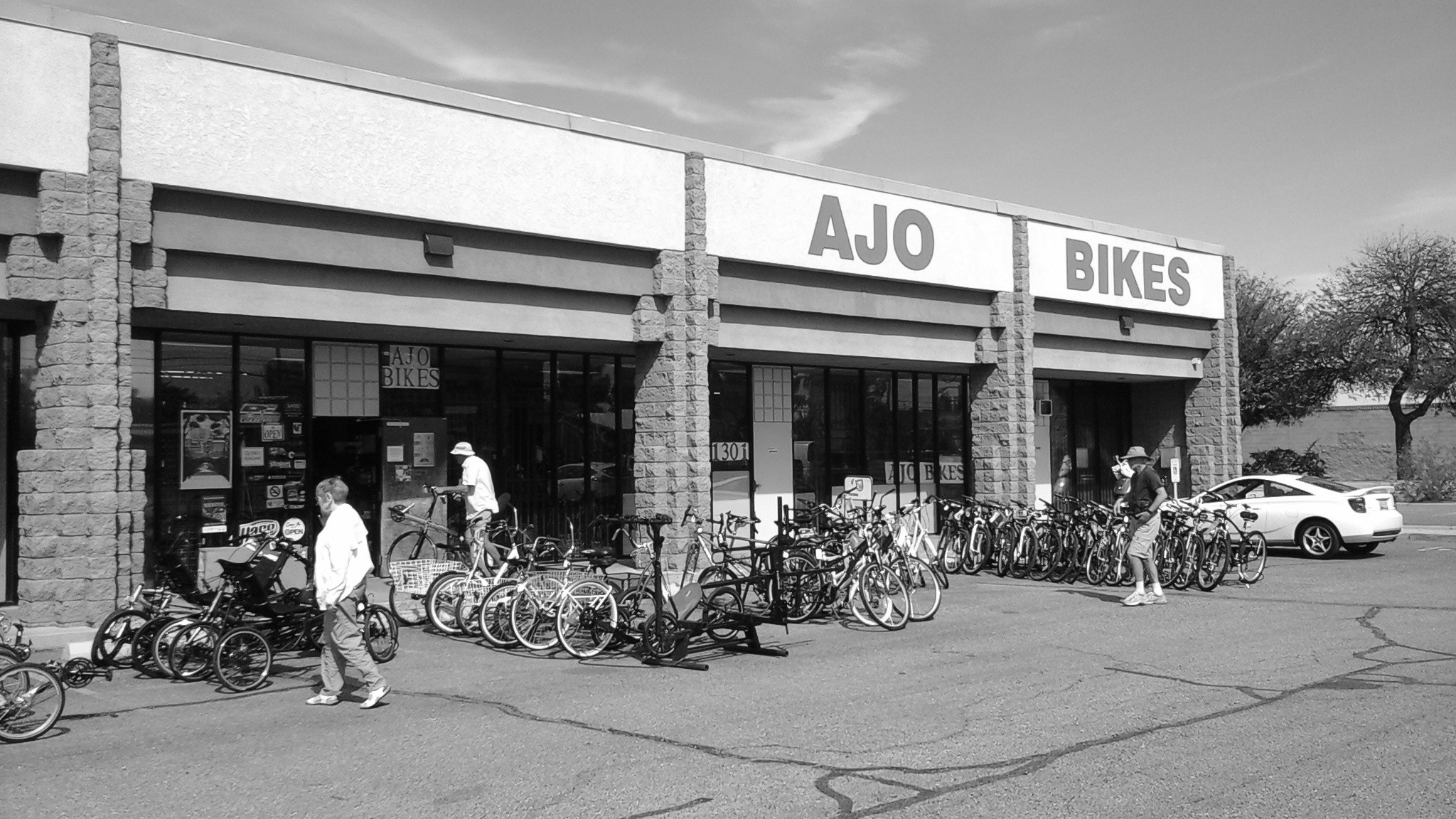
xmin=0 ymin=481 xmax=1271 ymax=745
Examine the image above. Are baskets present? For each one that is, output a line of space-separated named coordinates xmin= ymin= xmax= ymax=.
xmin=460 ymin=578 xmax=523 ymax=606
xmin=389 ymin=558 xmax=462 ymax=595
xmin=527 ymin=570 xmax=607 ymax=602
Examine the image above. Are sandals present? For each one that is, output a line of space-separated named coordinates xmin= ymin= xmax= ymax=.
xmin=359 ymin=685 xmax=391 ymax=708
xmin=306 ymin=694 xmax=339 ymax=705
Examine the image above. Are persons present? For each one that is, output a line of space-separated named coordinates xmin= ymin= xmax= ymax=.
xmin=306 ymin=477 xmax=392 ymax=708
xmin=434 ymin=442 xmax=504 ymax=577
xmin=1112 ymin=446 xmax=1167 ymax=605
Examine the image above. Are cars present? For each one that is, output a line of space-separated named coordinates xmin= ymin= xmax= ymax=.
xmin=548 ymin=462 xmax=621 ymax=505
xmin=1157 ymin=473 xmax=1404 ymax=560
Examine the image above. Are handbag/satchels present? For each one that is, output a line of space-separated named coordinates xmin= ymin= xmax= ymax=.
xmin=1133 ymin=500 xmax=1150 ymax=520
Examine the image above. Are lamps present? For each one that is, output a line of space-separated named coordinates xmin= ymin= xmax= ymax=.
xmin=1119 ymin=313 xmax=1135 ymax=330
xmin=422 ymin=232 xmax=454 ymax=256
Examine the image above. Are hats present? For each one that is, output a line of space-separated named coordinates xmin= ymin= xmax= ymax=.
xmin=1120 ymin=446 xmax=1152 ymax=462
xmin=450 ymin=441 xmax=475 ymax=456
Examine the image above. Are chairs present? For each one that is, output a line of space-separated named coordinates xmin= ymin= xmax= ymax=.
xmin=162 ymin=565 xmax=241 ymax=609
xmin=1269 ymin=486 xmax=1285 ymax=496
xmin=214 ymin=557 xmax=311 ymax=618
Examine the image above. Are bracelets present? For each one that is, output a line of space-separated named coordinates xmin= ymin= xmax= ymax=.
xmin=1146 ymin=509 xmax=1154 ymax=515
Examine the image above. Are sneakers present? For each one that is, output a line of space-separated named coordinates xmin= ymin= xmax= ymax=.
xmin=1143 ymin=591 xmax=1168 ymax=604
xmin=1123 ymin=591 xmax=1147 ymax=605
